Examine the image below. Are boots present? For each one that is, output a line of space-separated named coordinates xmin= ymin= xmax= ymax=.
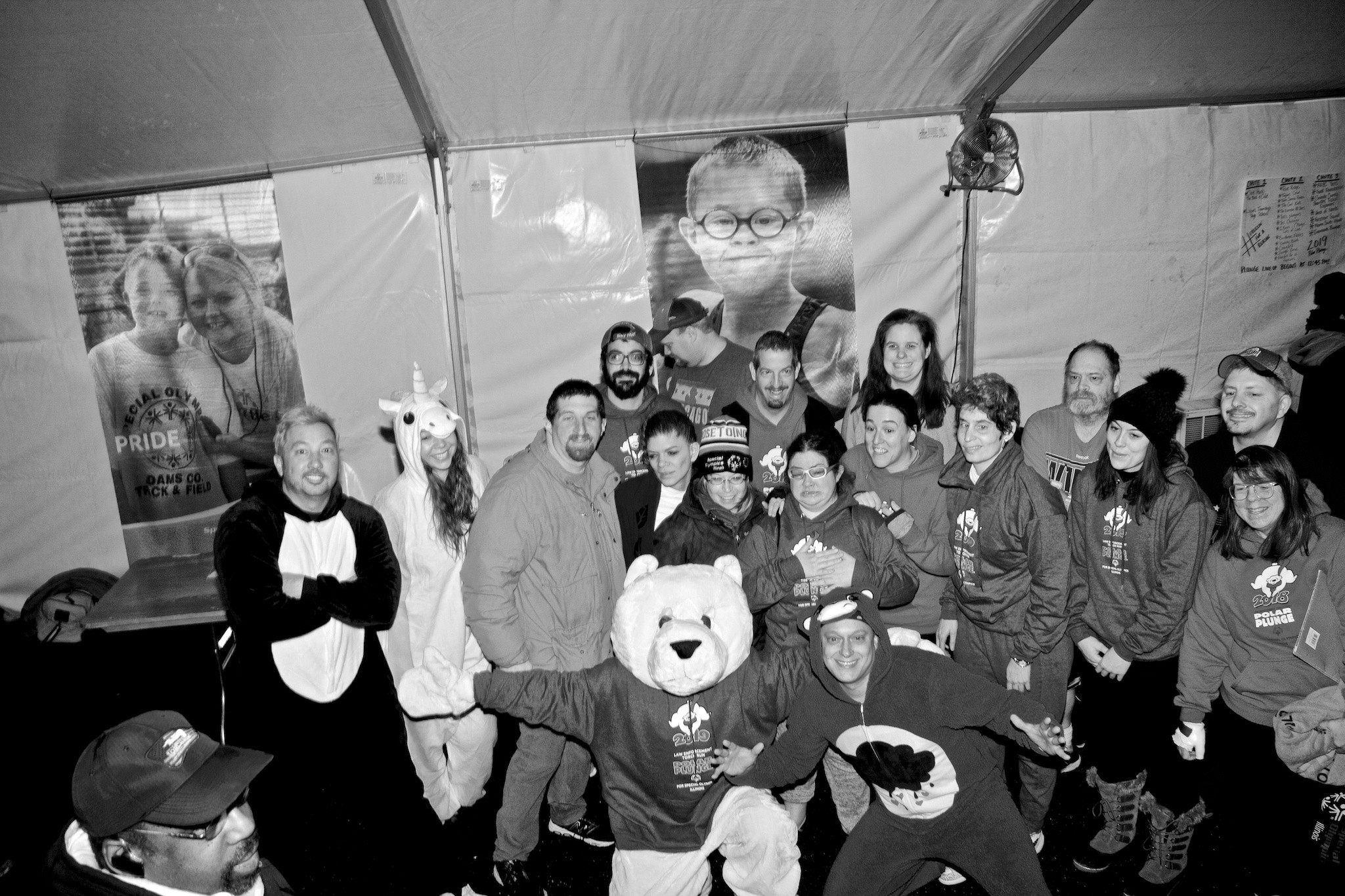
xmin=1072 ymin=769 xmax=1147 ymax=872
xmin=1122 ymin=790 xmax=1206 ymax=896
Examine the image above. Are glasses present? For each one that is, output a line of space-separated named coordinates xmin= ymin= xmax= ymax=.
xmin=693 ymin=201 xmax=802 ymax=242
xmin=1229 ymin=482 xmax=1278 ymax=501
xmin=124 ymin=789 xmax=250 ymax=848
xmin=787 ymin=464 xmax=837 ymax=479
xmin=707 ymin=476 xmax=746 ymax=485
xmin=607 ymin=351 xmax=646 ymax=365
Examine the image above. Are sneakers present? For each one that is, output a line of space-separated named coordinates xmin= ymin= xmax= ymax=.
xmin=549 ymin=812 xmax=616 ymax=847
xmin=493 ymin=857 xmax=547 ymax=896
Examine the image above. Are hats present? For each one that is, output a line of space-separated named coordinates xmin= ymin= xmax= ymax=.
xmin=1109 ymin=368 xmax=1187 ymax=453
xmin=648 ymin=298 xmax=709 ymax=342
xmin=69 ymin=709 xmax=275 ymax=838
xmin=1218 ymin=346 xmax=1291 ymax=392
xmin=601 ymin=321 xmax=652 ymax=353
xmin=695 ymin=414 xmax=753 ymax=481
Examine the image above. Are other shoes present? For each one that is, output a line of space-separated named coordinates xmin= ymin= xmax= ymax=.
xmin=939 ymin=865 xmax=966 ymax=885
xmin=1031 ymin=831 xmax=1045 ymax=854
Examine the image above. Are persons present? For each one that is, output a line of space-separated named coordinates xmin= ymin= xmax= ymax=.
xmin=1288 ymin=273 xmax=1345 ymax=518
xmin=841 ymin=307 xmax=961 ymax=465
xmin=213 ymin=406 xmax=469 ymax=896
xmin=710 ymin=580 xmax=1072 ymax=896
xmin=732 ymin=433 xmax=922 ymax=834
xmin=614 ymin=410 xmax=700 ymax=572
xmin=180 ymin=241 xmax=307 ymax=479
xmin=716 ymin=330 xmax=850 ymax=498
xmin=637 ymin=415 xmax=790 ymax=653
xmin=368 ymin=361 xmax=498 ymax=896
xmin=647 ymin=296 xmax=756 ymax=444
xmin=834 ymin=338 xmax=1345 ymax=896
xmin=14 ymin=709 xmax=297 ymax=896
xmin=397 ymin=554 xmax=945 ymax=896
xmin=87 ymin=241 xmax=249 ymax=523
xmin=679 ymin=137 xmax=858 ymax=441
xmin=464 ymin=378 xmax=627 ymax=896
xmin=592 ymin=321 xmax=688 ymax=485
xmin=939 ymin=372 xmax=1070 ymax=885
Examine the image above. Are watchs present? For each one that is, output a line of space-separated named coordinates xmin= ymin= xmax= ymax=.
xmin=1012 ymin=657 xmax=1031 ymax=667
